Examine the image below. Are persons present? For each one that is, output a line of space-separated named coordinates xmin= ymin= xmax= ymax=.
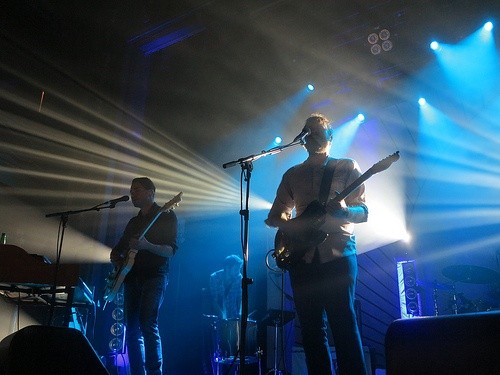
xmin=268 ymin=115 xmax=369 ymax=375
xmin=111 ymin=178 xmax=178 ymax=375
xmin=210 ymin=255 xmax=242 ymax=375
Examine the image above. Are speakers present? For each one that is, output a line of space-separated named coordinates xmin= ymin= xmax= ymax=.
xmin=384 ymin=308 xmax=500 ymax=375
xmin=0 ymin=324 xmax=112 ymax=375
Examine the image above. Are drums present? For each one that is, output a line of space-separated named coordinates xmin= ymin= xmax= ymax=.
xmin=215 ymin=318 xmax=261 ymax=365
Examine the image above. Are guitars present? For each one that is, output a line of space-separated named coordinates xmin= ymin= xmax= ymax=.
xmin=274 ymin=149 xmax=400 ymax=270
xmin=102 ymin=191 xmax=183 ymax=303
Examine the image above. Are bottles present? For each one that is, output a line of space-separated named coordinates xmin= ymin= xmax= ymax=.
xmin=0 ymin=232 xmax=7 ymax=245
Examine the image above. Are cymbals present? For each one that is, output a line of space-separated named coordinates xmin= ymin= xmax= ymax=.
xmin=442 ymin=265 xmax=499 ymax=283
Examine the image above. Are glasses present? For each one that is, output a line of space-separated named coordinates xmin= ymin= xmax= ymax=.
xmin=312 ymin=131 xmax=332 ymax=141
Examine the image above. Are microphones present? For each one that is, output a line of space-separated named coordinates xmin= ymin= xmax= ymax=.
xmin=105 ymin=196 xmax=129 ymax=205
xmin=291 ymin=128 xmax=312 ymax=146
